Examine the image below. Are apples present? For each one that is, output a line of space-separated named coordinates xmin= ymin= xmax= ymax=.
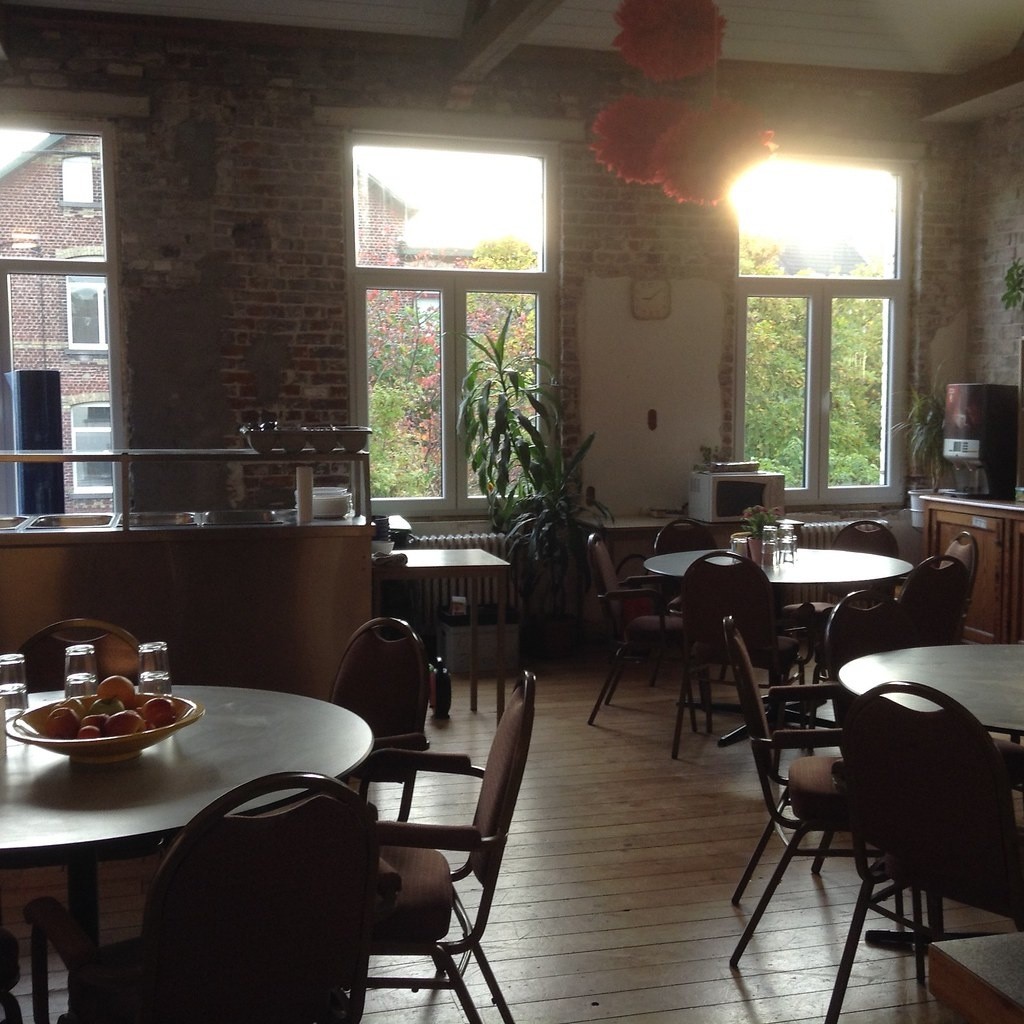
xmin=44 ymin=675 xmax=176 ymax=740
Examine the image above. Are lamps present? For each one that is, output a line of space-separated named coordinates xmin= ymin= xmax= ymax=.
xmin=590 ymin=0 xmax=787 ymax=207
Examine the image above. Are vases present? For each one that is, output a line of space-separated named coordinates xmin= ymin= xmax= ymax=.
xmin=747 ymin=537 xmax=780 ymax=564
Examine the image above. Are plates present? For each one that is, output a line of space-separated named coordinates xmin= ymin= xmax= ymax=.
xmin=294 ymin=487 xmax=353 ymax=518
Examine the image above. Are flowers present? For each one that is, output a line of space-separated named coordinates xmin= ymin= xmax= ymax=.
xmin=740 ymin=504 xmax=785 ymax=540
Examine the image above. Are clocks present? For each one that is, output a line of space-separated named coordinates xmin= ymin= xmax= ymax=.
xmin=629 ymin=278 xmax=673 ymax=320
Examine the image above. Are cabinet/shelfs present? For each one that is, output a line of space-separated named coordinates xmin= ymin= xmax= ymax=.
xmin=918 ymin=494 xmax=1024 ymax=645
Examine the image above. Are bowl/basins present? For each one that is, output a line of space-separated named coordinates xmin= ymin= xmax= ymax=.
xmin=5 ymin=692 xmax=205 ymax=765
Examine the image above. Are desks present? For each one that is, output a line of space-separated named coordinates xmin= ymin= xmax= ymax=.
xmin=573 ymin=513 xmax=805 ymax=632
xmin=0 ymin=685 xmax=374 ymax=949
xmin=643 ymin=548 xmax=914 ymax=746
xmin=372 ymin=547 xmax=511 ymax=727
xmin=837 ymin=644 xmax=1024 ymax=954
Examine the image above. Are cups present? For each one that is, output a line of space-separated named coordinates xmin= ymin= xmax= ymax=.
xmin=761 ymin=525 xmax=798 ymax=565
xmin=0 ymin=683 xmax=28 ymax=711
xmin=64 ymin=673 xmax=98 ymax=698
xmin=139 ymin=642 xmax=170 ymax=673
xmin=139 ymin=670 xmax=171 ymax=694
xmin=64 ymin=645 xmax=96 ymax=674
xmin=0 ymin=654 xmax=26 ymax=684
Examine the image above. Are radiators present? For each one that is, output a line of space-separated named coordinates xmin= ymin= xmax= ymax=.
xmin=783 ymin=519 xmax=892 ymax=604
xmin=406 ymin=530 xmax=550 ymax=635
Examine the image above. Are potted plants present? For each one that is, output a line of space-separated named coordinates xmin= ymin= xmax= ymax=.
xmin=888 ymin=355 xmax=959 ymax=532
xmin=456 ymin=308 xmax=619 ymax=662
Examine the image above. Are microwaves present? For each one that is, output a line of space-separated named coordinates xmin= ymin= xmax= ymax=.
xmin=687 ymin=471 xmax=786 ymax=524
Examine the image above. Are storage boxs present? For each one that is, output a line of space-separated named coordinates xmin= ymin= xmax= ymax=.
xmin=434 ymin=600 xmax=521 ymax=671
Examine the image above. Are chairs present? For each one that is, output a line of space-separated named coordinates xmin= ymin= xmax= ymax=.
xmin=22 ymin=770 xmax=380 ymax=1024
xmin=587 ymin=518 xmax=1024 ymax=1024
xmin=351 ymin=671 xmax=538 ymax=1024
xmin=329 ymin=616 xmax=432 ymax=822
xmin=16 ymin=617 xmax=142 ymax=694
